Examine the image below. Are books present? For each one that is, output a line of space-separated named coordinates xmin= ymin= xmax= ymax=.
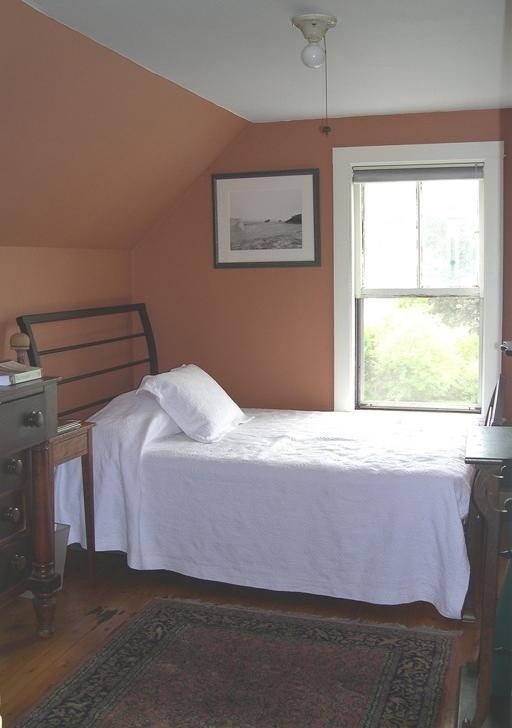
xmin=0 ymin=360 xmax=44 ymax=386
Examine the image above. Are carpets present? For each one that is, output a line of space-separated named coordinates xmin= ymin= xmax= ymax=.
xmin=14 ymin=596 xmax=462 ymax=728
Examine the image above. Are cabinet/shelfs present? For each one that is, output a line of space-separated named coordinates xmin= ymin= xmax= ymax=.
xmin=1 ymin=377 xmax=65 ymax=643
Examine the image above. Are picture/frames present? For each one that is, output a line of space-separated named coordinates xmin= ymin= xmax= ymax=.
xmin=212 ymin=167 xmax=322 ymax=267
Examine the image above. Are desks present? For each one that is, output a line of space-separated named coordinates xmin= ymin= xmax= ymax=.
xmin=32 ymin=422 xmax=100 ymax=585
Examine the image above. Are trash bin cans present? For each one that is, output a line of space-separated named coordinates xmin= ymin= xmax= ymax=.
xmin=19 ymin=522 xmax=71 ymax=595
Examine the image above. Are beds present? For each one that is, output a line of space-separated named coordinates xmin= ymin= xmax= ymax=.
xmin=16 ymin=303 xmax=483 ymax=594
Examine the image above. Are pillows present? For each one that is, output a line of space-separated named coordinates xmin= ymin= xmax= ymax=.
xmin=137 ymin=360 xmax=250 ymax=447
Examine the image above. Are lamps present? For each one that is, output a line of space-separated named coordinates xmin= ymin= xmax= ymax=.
xmin=291 ymin=12 xmax=338 ymax=70
